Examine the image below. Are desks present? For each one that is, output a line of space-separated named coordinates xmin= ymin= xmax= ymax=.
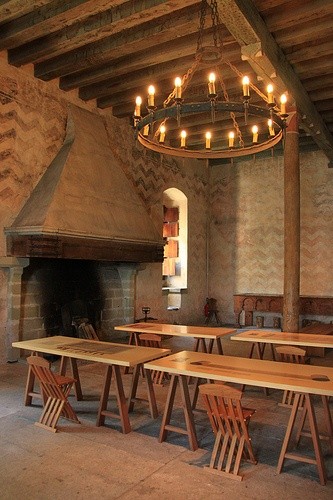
xmin=230 ymin=328 xmax=333 ymax=396
xmin=11 ymin=335 xmax=173 ymax=434
xmin=143 ymin=349 xmax=333 ymax=486
xmin=114 ymin=322 xmax=237 ymax=385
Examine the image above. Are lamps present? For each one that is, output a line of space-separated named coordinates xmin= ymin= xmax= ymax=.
xmin=132 ymin=1 xmax=289 ymax=160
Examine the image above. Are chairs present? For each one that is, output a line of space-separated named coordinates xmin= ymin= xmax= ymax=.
xmin=25 ymin=356 xmax=82 ymax=433
xmin=197 ymin=384 xmax=256 ymax=482
xmin=275 ymin=346 xmax=310 ymax=410
xmin=137 ymin=334 xmax=172 ymax=386
